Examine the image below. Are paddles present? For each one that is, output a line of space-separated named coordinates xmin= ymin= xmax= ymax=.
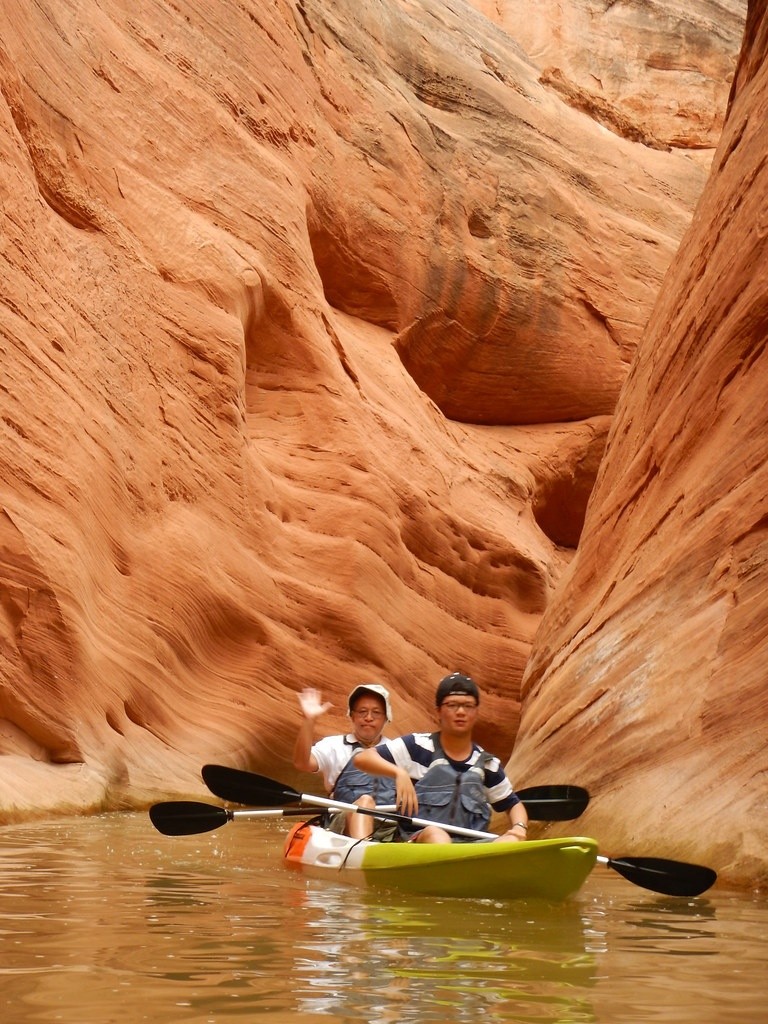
xmin=200 ymin=763 xmax=717 ymax=897
xmin=147 ymin=781 xmax=590 ymax=838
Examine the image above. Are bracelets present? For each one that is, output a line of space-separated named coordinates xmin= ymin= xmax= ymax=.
xmin=512 ymin=822 xmax=528 ymax=830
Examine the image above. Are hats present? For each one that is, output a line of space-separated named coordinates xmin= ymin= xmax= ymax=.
xmin=435 ymin=671 xmax=479 ymax=707
xmin=348 ymin=684 xmax=392 ymax=722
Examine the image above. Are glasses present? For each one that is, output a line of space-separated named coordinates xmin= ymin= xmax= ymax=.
xmin=439 ymin=701 xmax=476 ymax=711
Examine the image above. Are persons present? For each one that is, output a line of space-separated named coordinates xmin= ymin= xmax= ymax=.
xmin=292 ymin=684 xmax=397 ymax=841
xmin=353 ymin=672 xmax=529 ymax=843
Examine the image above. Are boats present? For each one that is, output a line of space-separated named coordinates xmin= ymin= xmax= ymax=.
xmin=282 ymin=819 xmax=598 ymax=901
